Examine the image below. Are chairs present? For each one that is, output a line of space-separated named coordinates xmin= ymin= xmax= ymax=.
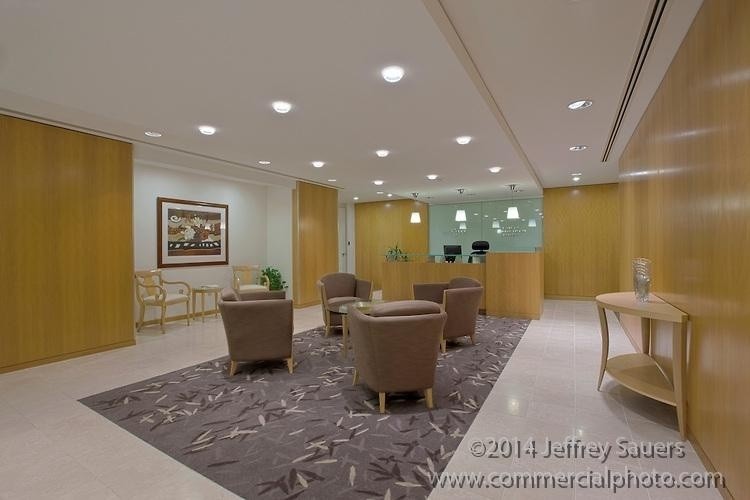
xmin=467 ymin=241 xmax=489 ymax=263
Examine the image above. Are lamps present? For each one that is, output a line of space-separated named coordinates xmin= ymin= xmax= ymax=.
xmin=410 ymin=185 xmax=519 ymax=224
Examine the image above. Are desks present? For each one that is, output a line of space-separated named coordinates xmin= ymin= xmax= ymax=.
xmin=595 ymin=291 xmax=688 ymax=442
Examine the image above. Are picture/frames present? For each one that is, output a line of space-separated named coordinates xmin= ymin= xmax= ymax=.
xmin=156 ymin=197 xmax=229 ymax=268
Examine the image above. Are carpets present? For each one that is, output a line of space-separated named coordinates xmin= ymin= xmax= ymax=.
xmin=77 ymin=315 xmax=532 ymax=500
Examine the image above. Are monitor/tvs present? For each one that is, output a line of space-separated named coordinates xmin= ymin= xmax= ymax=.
xmin=444 ymin=245 xmax=462 ymax=263
